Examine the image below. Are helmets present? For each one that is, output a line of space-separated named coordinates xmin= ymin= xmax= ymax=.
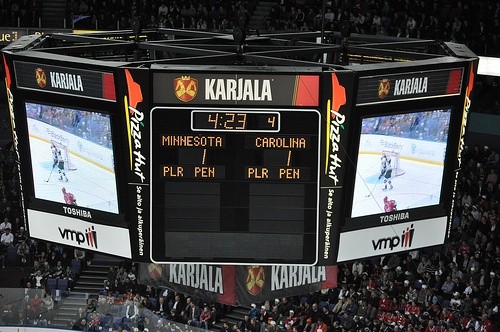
xmin=250 ymin=194 xmax=500 ymax=332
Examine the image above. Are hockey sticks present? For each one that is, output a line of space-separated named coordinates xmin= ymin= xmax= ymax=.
xmin=365 ymin=174 xmax=383 ymax=198
xmin=44 ymin=169 xmax=52 ymax=183
xmin=66 ymin=148 xmax=77 ymax=171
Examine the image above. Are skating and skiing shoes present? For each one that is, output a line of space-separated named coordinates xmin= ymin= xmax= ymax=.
xmin=382 ymin=186 xmax=393 ymax=191
xmin=58 ymin=177 xmax=68 ymax=182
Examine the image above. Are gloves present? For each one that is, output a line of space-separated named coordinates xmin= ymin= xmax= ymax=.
xmin=53 ymin=164 xmax=55 ymax=168
xmin=378 ymin=175 xmax=382 ymax=180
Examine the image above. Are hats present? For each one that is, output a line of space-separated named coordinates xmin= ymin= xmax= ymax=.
xmin=20 ymin=227 xmax=24 ymax=229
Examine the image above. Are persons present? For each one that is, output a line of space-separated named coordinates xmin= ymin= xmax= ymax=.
xmin=0 ymin=141 xmax=499 ymax=331
xmin=0 ymin=0 xmax=500 ymax=332
xmin=0 ymin=0 xmax=500 ymax=56
xmin=27 ymin=103 xmax=111 ymax=207
xmin=362 ymin=112 xmax=449 ymax=215
